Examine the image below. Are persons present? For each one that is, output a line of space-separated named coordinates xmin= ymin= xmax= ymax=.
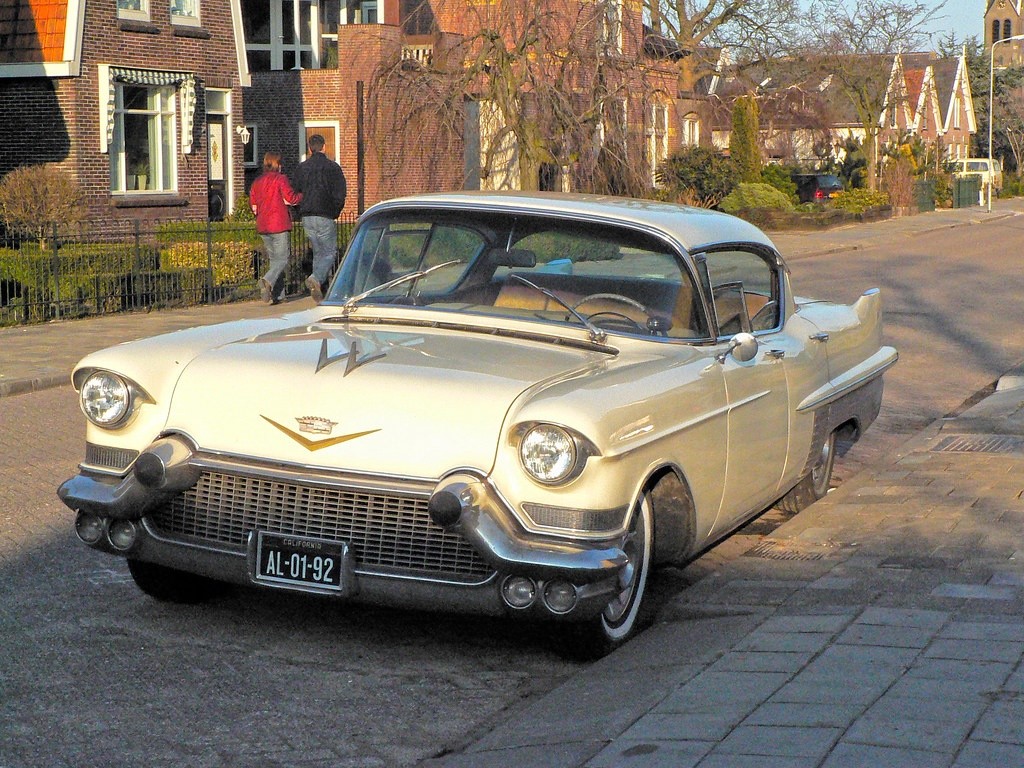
xmin=250 ymin=149 xmax=304 ymax=310
xmin=293 ymin=132 xmax=348 ymax=304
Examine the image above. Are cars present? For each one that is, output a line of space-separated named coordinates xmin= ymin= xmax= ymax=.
xmin=57 ymin=188 xmax=899 ymax=658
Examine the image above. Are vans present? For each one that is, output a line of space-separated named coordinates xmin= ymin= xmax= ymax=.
xmin=949 ymin=159 xmax=1003 ymax=192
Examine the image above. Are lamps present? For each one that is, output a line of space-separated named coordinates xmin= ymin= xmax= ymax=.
xmin=236 ymin=125 xmax=250 ymax=145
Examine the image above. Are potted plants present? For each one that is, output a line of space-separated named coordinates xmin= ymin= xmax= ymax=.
xmin=127 ymin=163 xmax=149 ymax=190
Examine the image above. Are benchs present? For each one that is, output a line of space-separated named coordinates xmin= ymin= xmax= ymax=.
xmin=492 ymin=272 xmax=772 ymax=337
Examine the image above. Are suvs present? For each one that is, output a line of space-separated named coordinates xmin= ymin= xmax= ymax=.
xmin=792 ymin=175 xmax=844 ymax=205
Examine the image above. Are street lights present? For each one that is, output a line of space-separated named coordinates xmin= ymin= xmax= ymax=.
xmin=988 ymin=33 xmax=1024 ymax=213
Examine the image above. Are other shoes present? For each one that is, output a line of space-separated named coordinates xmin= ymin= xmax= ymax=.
xmin=305 ymin=277 xmax=323 ymax=303
xmin=272 ymin=298 xmax=289 ymax=304
xmin=258 ymin=278 xmax=272 ymax=302
